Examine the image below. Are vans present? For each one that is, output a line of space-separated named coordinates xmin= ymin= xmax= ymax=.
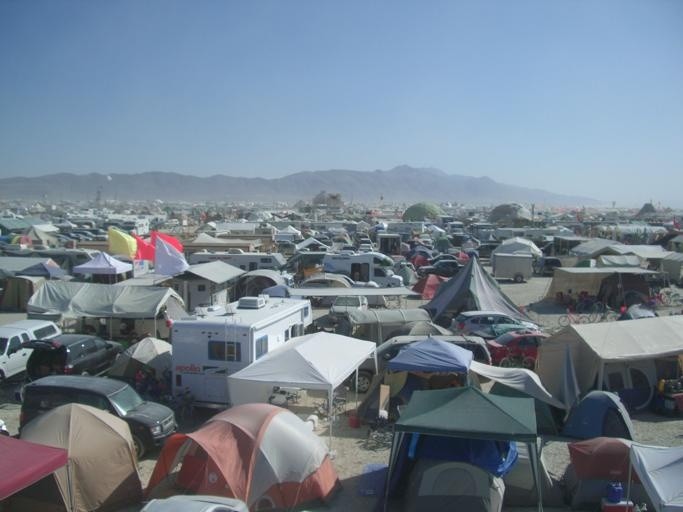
xmin=138 ymin=495 xmax=250 ymax=512
xmin=0 ymin=320 xmax=61 ymax=381
xmin=19 ymin=374 xmax=176 ymax=460
xmin=344 ymin=336 xmax=492 ymax=392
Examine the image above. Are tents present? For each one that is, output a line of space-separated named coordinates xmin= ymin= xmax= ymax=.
xmin=562 ymin=390 xmax=634 ymax=441
xmin=403 ymin=458 xmax=506 ymax=512
xmin=144 ymin=402 xmax=343 ymax=511
xmin=10 ymin=402 xmax=143 ymax=512
xmin=384 ymin=321 xmax=454 ymax=342
xmin=107 ymin=337 xmax=172 ymax=381
xmin=410 ymin=274 xmax=449 ymax=299
xmin=397 ymin=266 xmax=417 ymax=285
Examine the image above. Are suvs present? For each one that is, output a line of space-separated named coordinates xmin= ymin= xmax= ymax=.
xmin=450 ymin=310 xmax=540 ymax=337
xmin=533 ymin=257 xmax=561 ymax=276
xmin=21 ymin=334 xmax=124 ymax=381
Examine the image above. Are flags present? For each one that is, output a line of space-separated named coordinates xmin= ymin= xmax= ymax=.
xmin=132 ymin=231 xmax=152 ymax=260
xmin=155 ymin=235 xmax=190 ymax=275
xmin=109 ymin=228 xmax=137 ymax=260
xmin=150 ymin=230 xmax=183 ymax=260
xmin=418 ymin=253 xmax=534 ymax=327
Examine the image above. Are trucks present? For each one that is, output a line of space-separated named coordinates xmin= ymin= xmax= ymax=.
xmin=188 ymin=248 xmax=287 ymax=272
xmin=324 ymin=252 xmax=404 ymax=289
xmin=172 ymin=294 xmax=313 ymax=410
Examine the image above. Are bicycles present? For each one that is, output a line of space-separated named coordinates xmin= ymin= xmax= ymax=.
xmin=557 ymin=285 xmax=667 ymax=327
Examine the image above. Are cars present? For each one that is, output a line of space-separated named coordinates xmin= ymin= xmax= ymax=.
xmin=303 ymin=227 xmax=501 ymax=277
xmin=52 ymin=228 xmax=110 ymax=242
xmin=329 ymin=295 xmax=368 ymax=324
xmin=470 ymin=324 xmax=552 ymax=370
